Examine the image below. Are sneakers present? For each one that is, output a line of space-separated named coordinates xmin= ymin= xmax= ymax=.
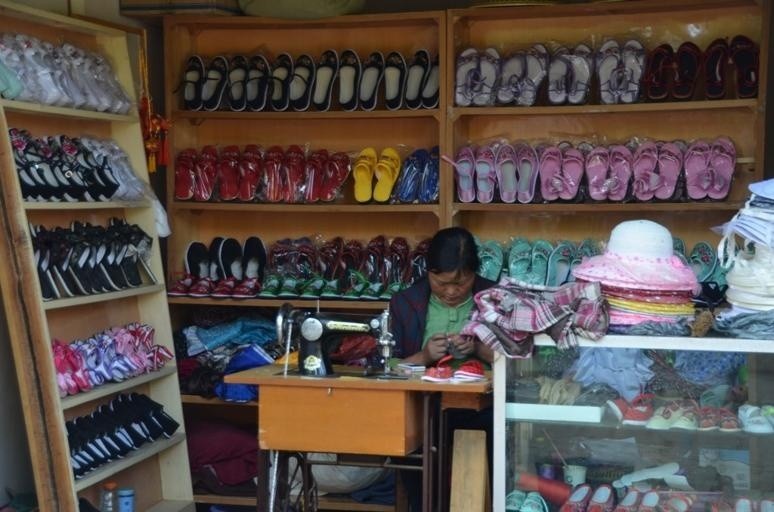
xmin=420 ymin=366 xmax=452 ymax=383
xmin=6 ymin=128 xmax=147 ymax=201
xmin=455 ymin=361 xmax=485 ymax=379
xmin=167 ymin=235 xmax=434 ymax=300
xmin=674 ymin=236 xmax=717 ymax=281
xmin=176 ymin=47 xmax=441 ymax=111
xmin=602 ymin=394 xmax=774 ymax=435
xmin=507 ymin=485 xmax=774 ymax=512
xmin=1 ymin=36 xmax=137 ymax=114
xmin=66 ymin=394 xmax=180 ymax=479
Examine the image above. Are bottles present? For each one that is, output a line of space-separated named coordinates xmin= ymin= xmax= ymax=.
xmin=98 ymin=481 xmax=119 ymax=512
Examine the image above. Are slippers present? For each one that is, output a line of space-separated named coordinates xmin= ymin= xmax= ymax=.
xmin=31 ymin=218 xmax=162 ymax=301
xmin=174 ymin=146 xmax=440 ymax=204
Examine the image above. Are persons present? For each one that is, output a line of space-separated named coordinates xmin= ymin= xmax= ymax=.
xmin=385 ymin=225 xmax=505 ymax=377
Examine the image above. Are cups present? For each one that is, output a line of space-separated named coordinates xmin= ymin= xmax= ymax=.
xmin=562 ymin=464 xmax=587 ymax=486
xmin=117 ymin=488 xmax=136 ymax=512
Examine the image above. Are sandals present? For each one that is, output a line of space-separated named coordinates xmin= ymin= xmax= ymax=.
xmin=455 ymin=135 xmax=737 ymax=204
xmin=52 ymin=323 xmax=176 ymax=400
xmin=474 ymin=237 xmax=601 ymax=288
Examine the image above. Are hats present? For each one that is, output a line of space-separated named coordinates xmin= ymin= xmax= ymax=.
xmin=570 ymin=221 xmax=699 ymax=327
xmin=712 ymin=179 xmax=774 ymax=314
